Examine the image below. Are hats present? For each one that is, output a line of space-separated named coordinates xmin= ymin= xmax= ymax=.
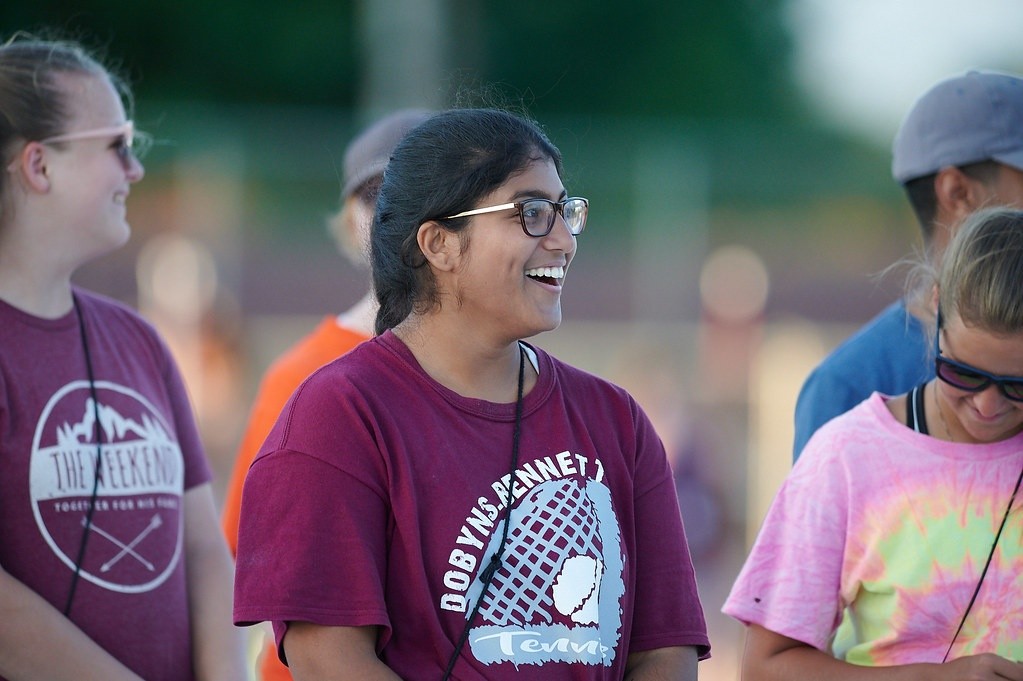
xmin=891 ymin=71 xmax=1022 ymax=184
xmin=341 ymin=109 xmax=431 ymax=195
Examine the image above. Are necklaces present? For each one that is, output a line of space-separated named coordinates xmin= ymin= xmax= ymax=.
xmin=934 ymin=380 xmax=954 ymax=443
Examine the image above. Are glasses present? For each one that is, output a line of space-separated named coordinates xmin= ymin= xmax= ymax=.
xmin=933 ymin=295 xmax=1023 ymax=402
xmin=7 ymin=119 xmax=135 ymax=174
xmin=416 ymin=197 xmax=589 ymax=237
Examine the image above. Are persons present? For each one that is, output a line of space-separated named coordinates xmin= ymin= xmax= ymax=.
xmin=0 ymin=43 xmax=252 ymax=681
xmin=220 ymin=110 xmax=433 ymax=681
xmin=793 ymin=70 xmax=1023 ymax=467
xmin=233 ymin=109 xmax=711 ymax=681
xmin=719 ymin=209 xmax=1023 ymax=681
